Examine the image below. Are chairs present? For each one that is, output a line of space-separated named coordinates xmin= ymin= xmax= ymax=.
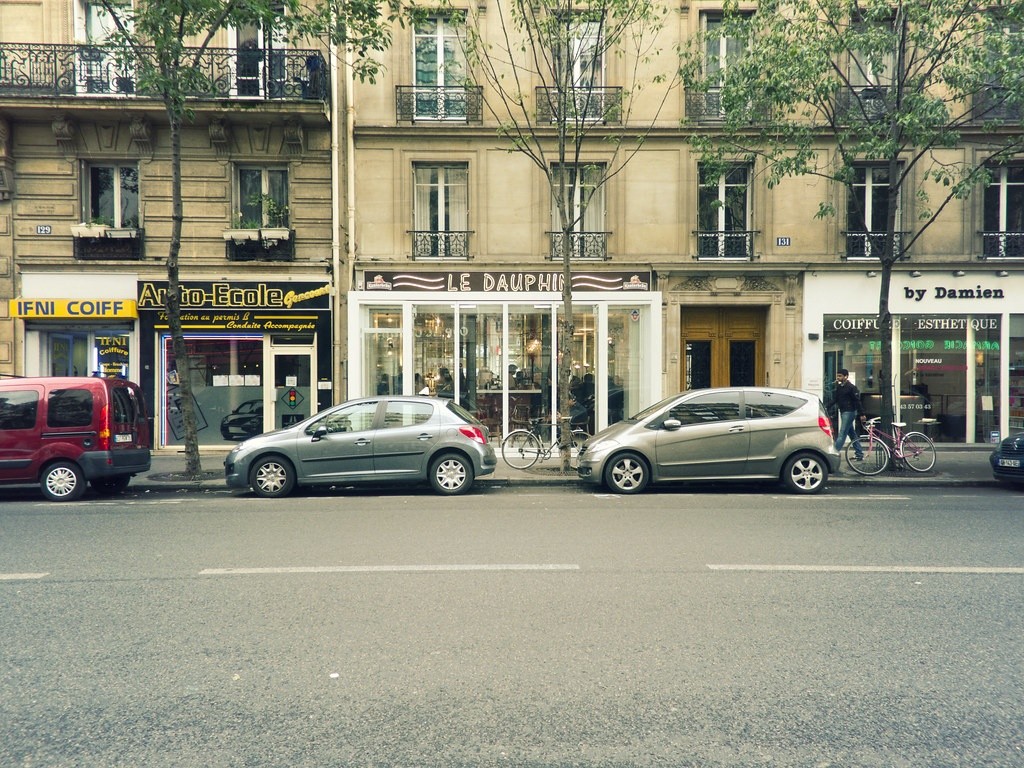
xmin=478 ymin=398 xmax=502 ymax=448
xmin=509 ymin=404 xmax=552 ymax=447
xmin=571 ymin=416 xmax=590 ymax=434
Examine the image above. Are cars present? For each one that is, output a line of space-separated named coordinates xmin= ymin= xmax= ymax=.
xmin=221 ymin=395 xmax=497 ymax=498
xmin=576 ymin=386 xmax=842 ymax=495
xmin=219 ymin=399 xmax=265 ymax=440
xmin=989 ymin=430 xmax=1024 ymax=484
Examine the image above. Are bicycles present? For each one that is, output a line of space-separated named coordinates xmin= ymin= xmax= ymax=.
xmin=845 ymin=414 xmax=938 ymax=475
xmin=500 ymin=415 xmax=593 ymax=472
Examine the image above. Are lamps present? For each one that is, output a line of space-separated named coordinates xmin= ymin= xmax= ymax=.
xmin=912 ymin=271 xmax=921 ymax=276
xmin=526 ymin=338 xmax=542 ymax=390
xmin=1000 ymin=271 xmax=1009 ymax=277
xmin=956 ymin=271 xmax=965 ymax=276
xmin=867 ymin=272 xmax=876 ymax=276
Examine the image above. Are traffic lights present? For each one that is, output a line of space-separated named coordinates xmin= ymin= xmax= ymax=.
xmin=289 ymin=390 xmax=296 ymax=407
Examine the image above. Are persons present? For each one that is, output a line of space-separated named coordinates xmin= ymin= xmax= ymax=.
xmin=438 ymin=367 xmax=454 ymax=391
xmin=509 ymin=364 xmax=518 ymax=389
xmin=377 ymin=373 xmax=390 ymax=395
xmin=547 ymin=373 xmax=624 ymax=440
xmin=825 ymin=369 xmax=866 ymax=462
xmin=415 ymin=373 xmax=429 ymax=392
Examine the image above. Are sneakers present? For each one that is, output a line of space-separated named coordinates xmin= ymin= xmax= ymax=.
xmin=849 ymin=457 xmax=862 ymax=461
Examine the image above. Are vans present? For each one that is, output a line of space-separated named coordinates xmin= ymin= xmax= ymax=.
xmin=0 ymin=371 xmax=152 ymax=501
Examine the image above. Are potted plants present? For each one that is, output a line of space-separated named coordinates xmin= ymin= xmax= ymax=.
xmin=247 ymin=192 xmax=291 ymax=240
xmin=73 ymin=38 xmax=107 ymax=61
xmin=70 ymin=215 xmax=110 ymax=238
xmin=220 ymin=206 xmax=259 ymax=240
xmin=104 ymin=219 xmax=138 ymax=238
